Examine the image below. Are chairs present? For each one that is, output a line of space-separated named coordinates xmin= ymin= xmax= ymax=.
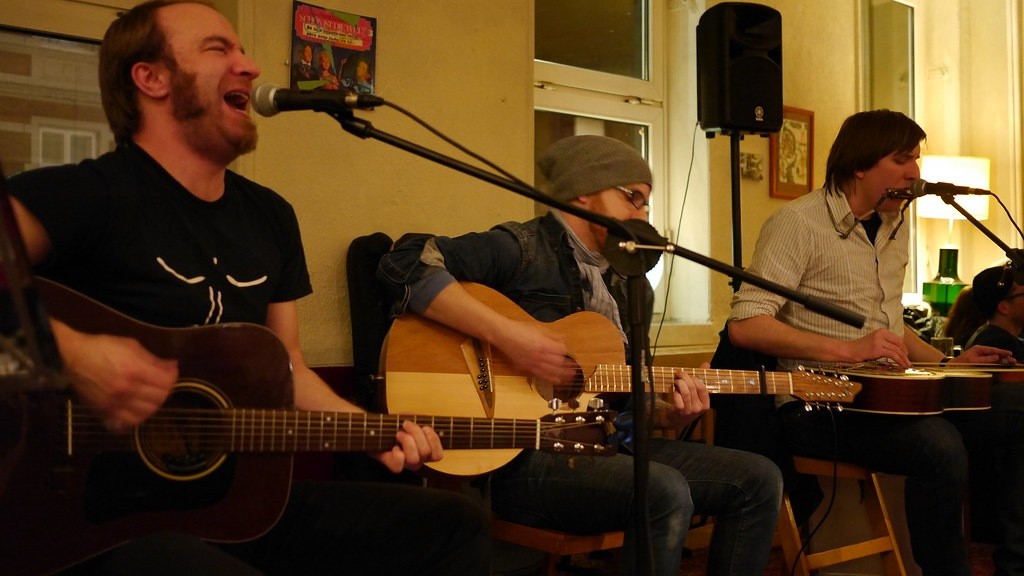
xmin=479 ymin=505 xmax=627 ymax=576
xmin=751 ymin=454 xmax=912 ymax=576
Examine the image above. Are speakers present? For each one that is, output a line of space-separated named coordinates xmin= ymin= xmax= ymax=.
xmin=697 ymin=2 xmax=784 ymax=140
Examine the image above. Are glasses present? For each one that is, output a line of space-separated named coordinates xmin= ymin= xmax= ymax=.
xmin=612 ymin=185 xmax=649 ymax=213
xmin=1005 ymin=292 xmax=1023 ymax=302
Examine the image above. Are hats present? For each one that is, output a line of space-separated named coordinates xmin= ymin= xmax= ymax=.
xmin=541 ymin=135 xmax=652 ymax=201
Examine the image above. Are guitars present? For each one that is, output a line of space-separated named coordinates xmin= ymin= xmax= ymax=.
xmin=367 ymin=279 xmax=865 ymax=475
xmin=777 ymin=357 xmax=1024 ymax=415
xmin=0 ymin=273 xmax=617 ymax=575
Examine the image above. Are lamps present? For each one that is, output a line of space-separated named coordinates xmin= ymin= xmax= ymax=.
xmin=912 ymin=154 xmax=992 ymax=315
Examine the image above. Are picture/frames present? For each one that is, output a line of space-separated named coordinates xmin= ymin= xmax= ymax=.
xmin=769 ymin=105 xmax=816 ymax=200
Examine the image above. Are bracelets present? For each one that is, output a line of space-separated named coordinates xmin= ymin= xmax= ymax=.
xmin=938 ymin=356 xmax=954 ymax=368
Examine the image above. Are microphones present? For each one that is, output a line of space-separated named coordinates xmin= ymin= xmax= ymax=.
xmin=910 ymin=180 xmax=989 ymax=198
xmin=251 ymin=80 xmax=383 ymax=118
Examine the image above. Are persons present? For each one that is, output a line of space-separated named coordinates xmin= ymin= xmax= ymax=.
xmin=961 ymin=265 xmax=1024 ymax=364
xmin=372 ymin=134 xmax=782 ymax=576
xmin=726 ymin=109 xmax=1024 ymax=576
xmin=940 ymin=285 xmax=987 ymax=353
xmin=0 ymin=0 xmax=494 ymax=576
